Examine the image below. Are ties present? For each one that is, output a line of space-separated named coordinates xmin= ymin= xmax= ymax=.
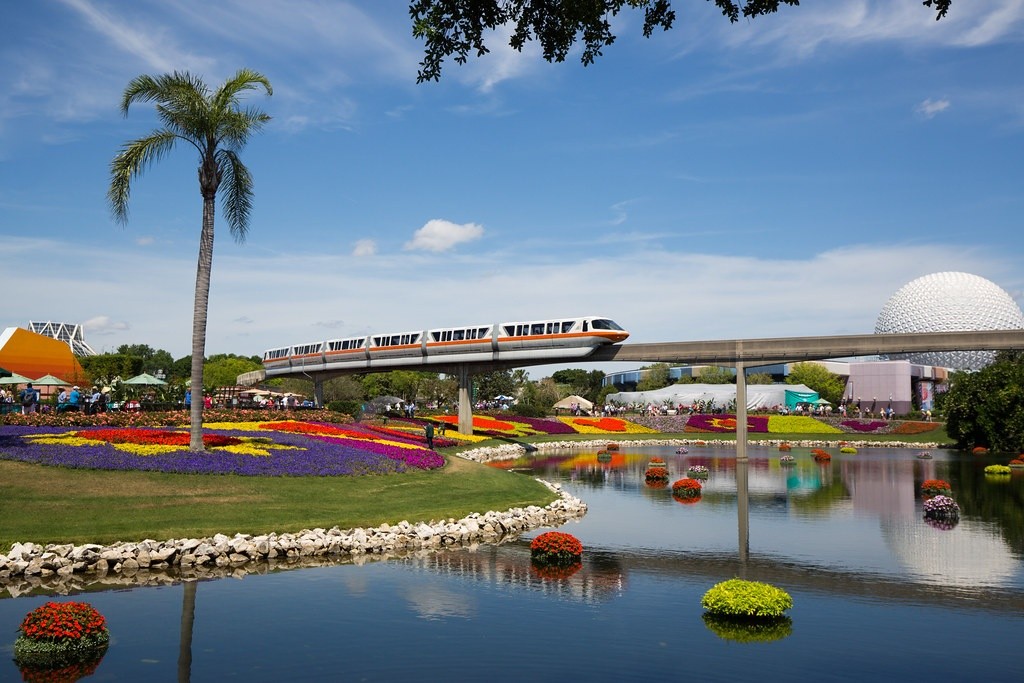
xmin=257 ymin=398 xmax=258 ymax=402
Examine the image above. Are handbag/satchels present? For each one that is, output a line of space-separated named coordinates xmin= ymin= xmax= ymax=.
xmin=438 ymin=427 xmax=442 ymax=432
xmin=274 ymin=401 xmax=279 ymax=406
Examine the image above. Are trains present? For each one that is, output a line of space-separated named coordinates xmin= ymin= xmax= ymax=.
xmin=261 ymin=316 xmax=630 ymax=370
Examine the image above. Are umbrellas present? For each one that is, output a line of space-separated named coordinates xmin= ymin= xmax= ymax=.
xmin=812 ymin=397 xmax=832 ymax=407
xmin=123 ymin=371 xmax=169 ymax=396
xmin=494 ymin=394 xmax=514 ymax=401
xmin=0 ymin=369 xmax=72 ymax=402
xmin=239 ymin=386 xmax=306 ymax=401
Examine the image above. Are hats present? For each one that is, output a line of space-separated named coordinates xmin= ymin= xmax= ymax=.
xmin=269 ymin=397 xmax=273 ymax=399
xmin=57 ymin=386 xmax=66 ymax=391
xmin=73 ymin=386 xmax=80 ymax=390
xmin=93 ymin=386 xmax=98 ymax=391
xmin=101 ymin=387 xmax=111 ymax=395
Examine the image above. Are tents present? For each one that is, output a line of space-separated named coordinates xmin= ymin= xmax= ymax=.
xmin=605 ymin=384 xmax=820 ymax=415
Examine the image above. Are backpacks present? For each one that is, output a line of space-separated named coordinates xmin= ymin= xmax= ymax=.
xmin=23 ymin=390 xmax=36 ymax=407
xmin=5 ymin=391 xmax=13 ymax=403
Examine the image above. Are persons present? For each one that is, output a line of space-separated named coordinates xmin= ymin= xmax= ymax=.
xmin=360 ymin=395 xmax=512 ymax=453
xmin=0 ymin=379 xmax=182 ymax=410
xmin=751 ymin=400 xmax=897 ymax=420
xmin=224 ymin=395 xmax=316 ymax=410
xmin=921 ymin=408 xmax=944 ymax=422
xmin=570 ymin=399 xmax=732 ymax=417
xmin=184 ymin=387 xmax=212 ymax=411
